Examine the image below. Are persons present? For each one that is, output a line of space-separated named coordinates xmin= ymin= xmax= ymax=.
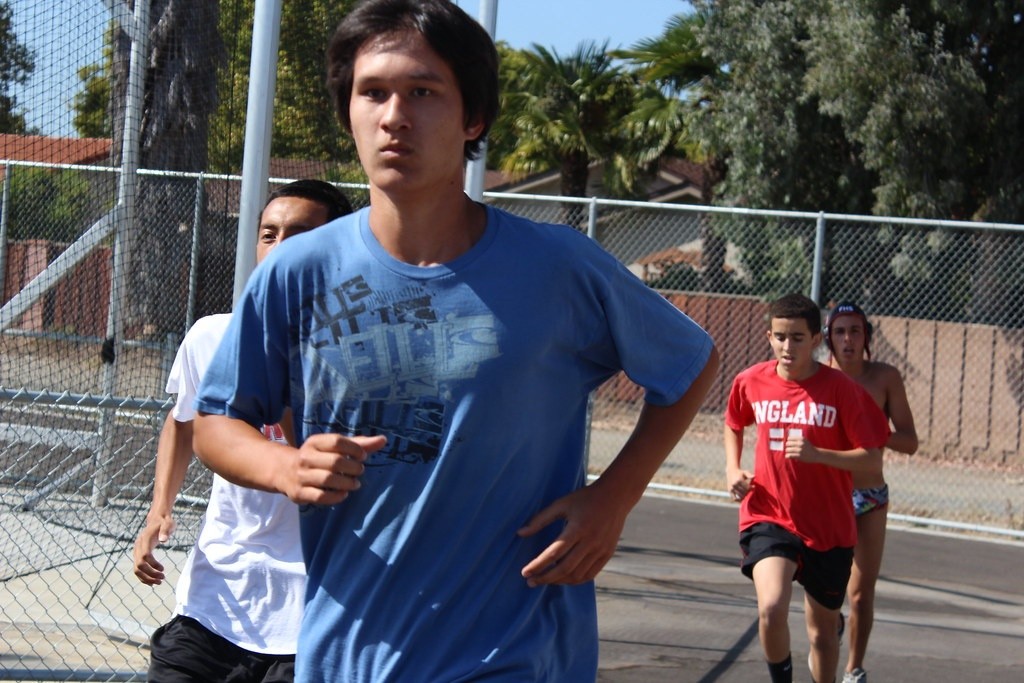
xmin=132 ymin=176 xmax=353 ymax=683
xmin=191 ymin=1 xmax=723 ymax=683
xmin=807 ymin=299 xmax=919 ymax=683
xmin=723 ymin=291 xmax=892 ymax=683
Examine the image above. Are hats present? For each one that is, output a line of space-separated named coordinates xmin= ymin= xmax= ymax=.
xmin=824 ymin=301 xmax=873 ymax=368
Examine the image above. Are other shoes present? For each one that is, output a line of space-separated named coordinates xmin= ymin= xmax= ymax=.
xmin=808 ymin=613 xmax=845 ymax=676
xmin=842 ymin=667 xmax=870 ymax=683
